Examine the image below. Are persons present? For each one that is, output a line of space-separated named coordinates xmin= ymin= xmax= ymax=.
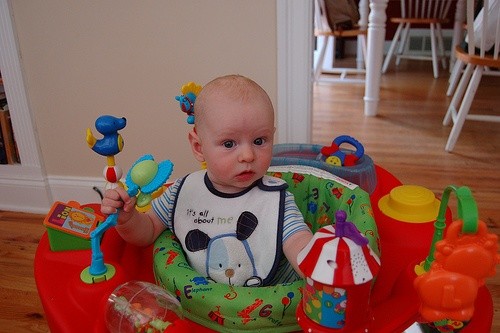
xmin=100 ymin=75 xmax=315 ymax=289
xmin=462 ymin=0 xmax=500 ymax=74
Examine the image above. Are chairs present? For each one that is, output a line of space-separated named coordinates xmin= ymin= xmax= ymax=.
xmin=312 ymin=0 xmax=500 ymax=152
